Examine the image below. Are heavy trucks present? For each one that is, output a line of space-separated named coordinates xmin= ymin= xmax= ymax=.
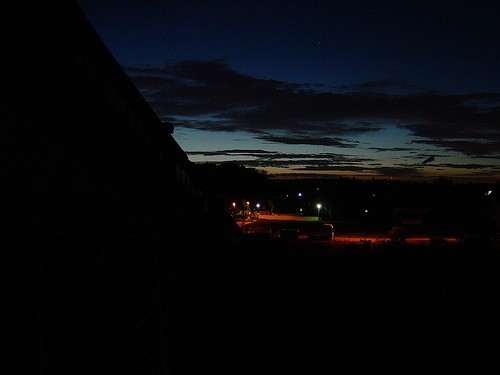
xmin=280 ymin=223 xmax=334 ymax=240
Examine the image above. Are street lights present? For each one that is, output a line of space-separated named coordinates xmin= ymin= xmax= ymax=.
xmin=317 ymin=204 xmax=322 ymax=222
xmin=233 ymin=203 xmax=236 ymax=217
